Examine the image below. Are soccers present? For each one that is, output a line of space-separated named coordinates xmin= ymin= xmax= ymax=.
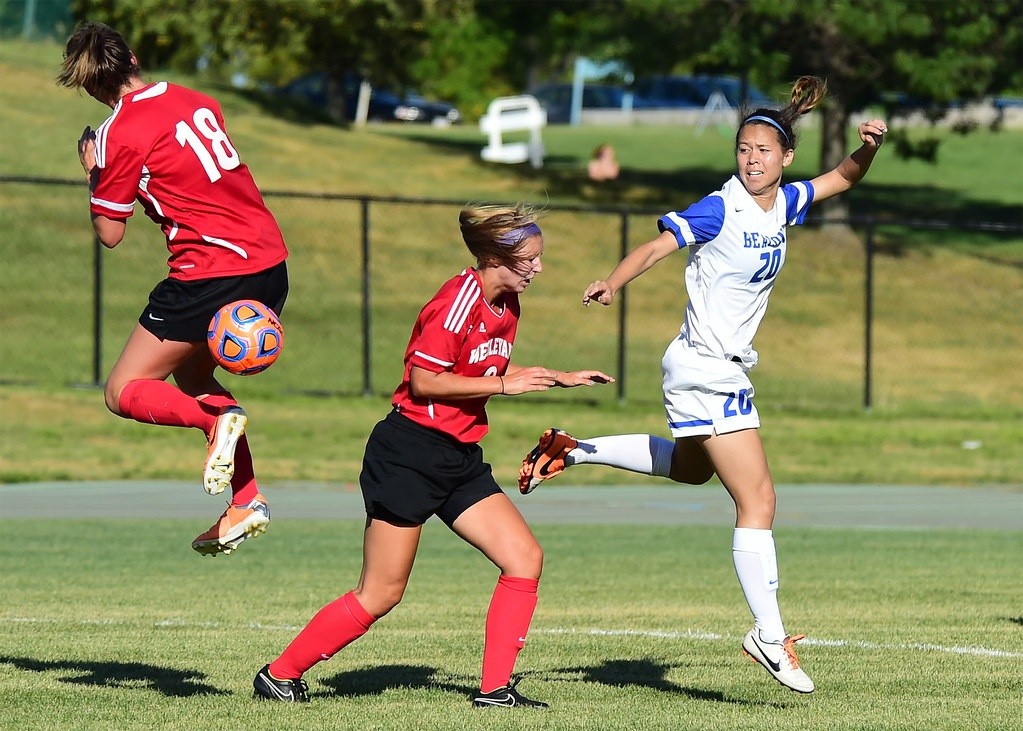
xmin=208 ymin=300 xmax=285 ymax=376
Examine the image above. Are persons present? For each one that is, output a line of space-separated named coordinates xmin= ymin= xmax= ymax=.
xmin=588 ymin=138 xmax=620 ymax=182
xmin=249 ymin=201 xmax=618 ymax=708
xmin=517 ymin=77 xmax=888 ymax=693
xmin=55 ymin=16 xmax=292 ymax=557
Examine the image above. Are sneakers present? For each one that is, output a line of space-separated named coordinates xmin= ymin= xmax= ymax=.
xmin=518 ymin=426 xmax=578 ymax=495
xmin=471 ymin=683 xmax=549 ymax=710
xmin=201 ymin=405 xmax=249 ymax=495
xmin=252 ymin=663 xmax=310 ymax=704
xmin=741 ymin=625 xmax=815 ymax=694
xmin=192 ymin=491 xmax=270 ymax=557
